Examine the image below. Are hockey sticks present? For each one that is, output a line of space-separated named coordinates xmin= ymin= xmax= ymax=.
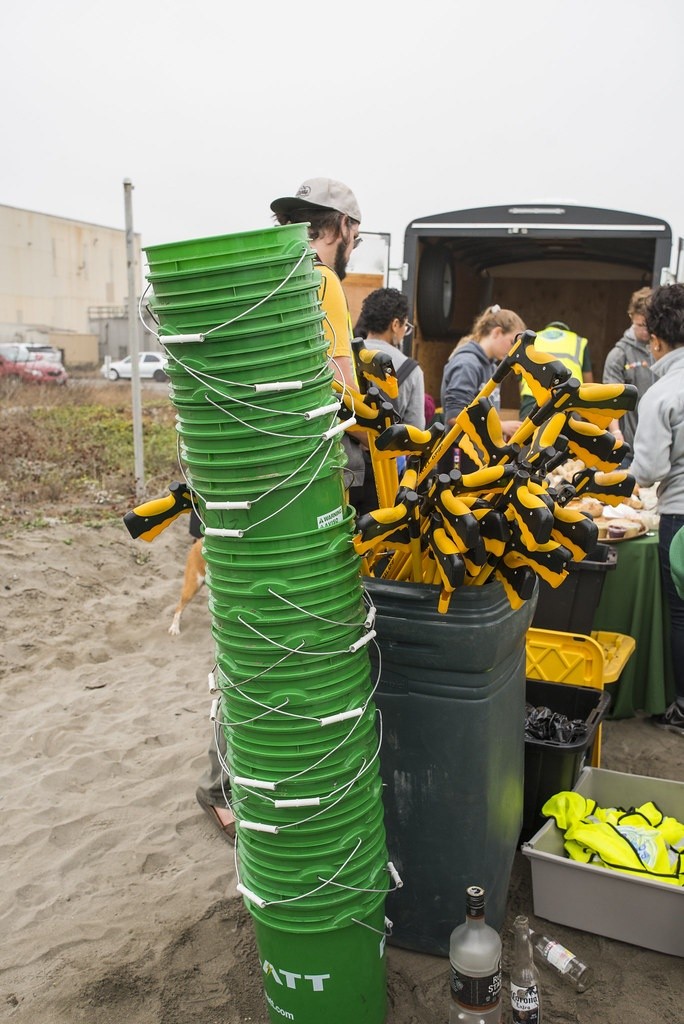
xmin=120 ymin=329 xmax=638 ymax=620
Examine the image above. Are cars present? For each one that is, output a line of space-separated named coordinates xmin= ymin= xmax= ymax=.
xmin=0 ymin=343 xmax=61 ymax=363
xmin=0 ymin=348 xmax=67 ymax=386
xmin=101 ymin=352 xmax=169 ymax=382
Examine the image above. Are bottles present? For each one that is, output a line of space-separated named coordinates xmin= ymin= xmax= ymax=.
xmin=447 ymin=886 xmax=503 ymax=1024
xmin=526 ymin=928 xmax=596 ymax=994
xmin=509 ymin=915 xmax=545 ymax=1024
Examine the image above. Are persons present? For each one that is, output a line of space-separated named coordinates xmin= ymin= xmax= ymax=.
xmin=515 ymin=321 xmax=593 ymax=425
xmin=603 ymin=286 xmax=658 ymax=469
xmin=440 ymin=303 xmax=525 ymax=443
xmin=354 ymin=288 xmax=435 ymax=516
xmin=195 ymin=177 xmax=365 ymax=845
xmin=628 ymin=283 xmax=684 ymax=735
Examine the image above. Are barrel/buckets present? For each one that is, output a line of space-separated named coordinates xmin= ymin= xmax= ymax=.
xmin=134 ymin=221 xmax=638 ymax=1023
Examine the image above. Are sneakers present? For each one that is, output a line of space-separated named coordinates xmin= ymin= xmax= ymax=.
xmin=650 ymin=699 xmax=684 ymax=736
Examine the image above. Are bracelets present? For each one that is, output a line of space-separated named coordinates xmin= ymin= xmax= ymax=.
xmin=611 ymin=429 xmax=622 ymax=436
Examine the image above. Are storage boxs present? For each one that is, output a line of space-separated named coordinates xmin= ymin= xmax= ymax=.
xmin=522 ymin=766 xmax=684 ymax=958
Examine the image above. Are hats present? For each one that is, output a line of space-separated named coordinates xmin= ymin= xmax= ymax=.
xmin=270 ymin=177 xmax=362 ymax=224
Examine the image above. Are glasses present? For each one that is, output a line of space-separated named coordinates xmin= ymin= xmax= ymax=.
xmin=403 ymin=320 xmax=415 ymax=336
xmin=353 ymin=237 xmax=363 ymax=249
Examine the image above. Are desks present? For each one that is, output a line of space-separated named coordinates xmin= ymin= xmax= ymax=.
xmin=588 ymin=529 xmax=679 ymax=720
